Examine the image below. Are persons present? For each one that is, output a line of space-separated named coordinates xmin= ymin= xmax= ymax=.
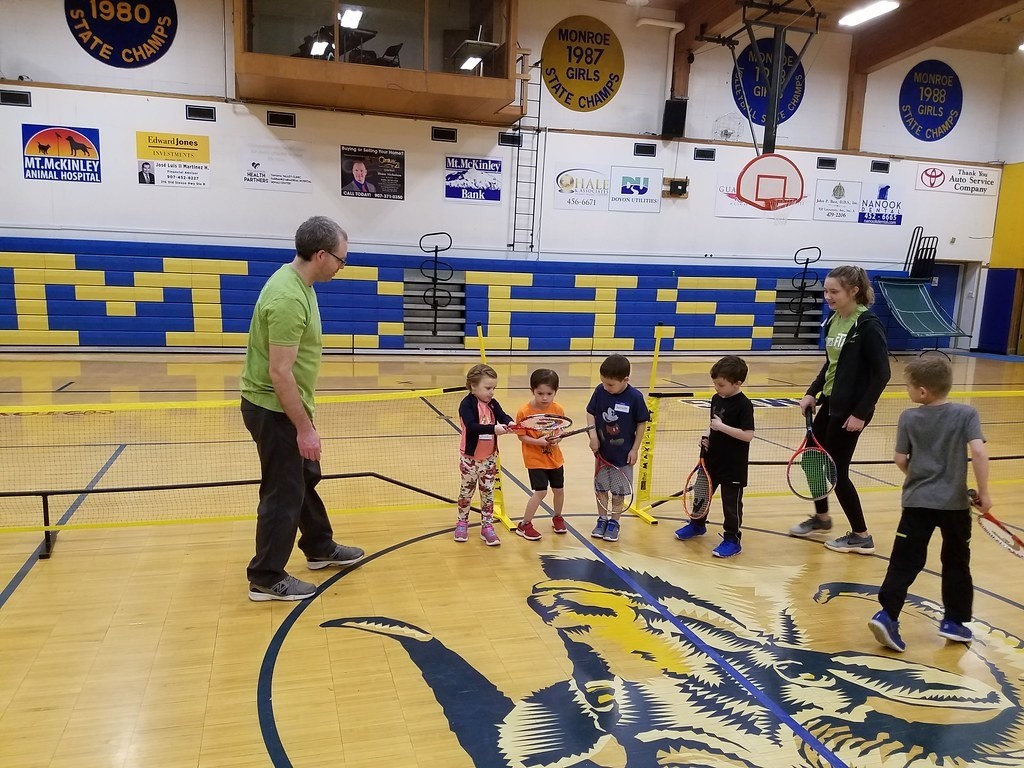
xmin=139 ymin=162 xmax=155 ymax=184
xmin=516 ymin=369 xmax=568 ymax=541
xmin=241 ymin=215 xmax=365 ymax=601
xmin=452 ymin=362 xmax=520 ymax=545
xmin=789 ymin=265 xmax=890 ymax=555
xmin=343 ymin=160 xmax=376 ymax=194
xmin=586 ymin=354 xmax=646 ymax=542
xmin=868 ymin=354 xmax=992 ymax=653
xmin=674 ymin=355 xmax=756 ymax=557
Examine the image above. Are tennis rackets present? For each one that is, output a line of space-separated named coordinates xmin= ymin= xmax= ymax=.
xmin=548 ymin=424 xmax=595 ymax=440
xmin=585 ymin=427 xmax=633 ymax=514
xmin=504 ymin=414 xmax=573 ymax=430
xmin=683 ymin=436 xmax=712 ymax=519
xmin=967 ymin=489 xmax=1024 ymax=558
xmin=787 ymin=407 xmax=838 ymax=502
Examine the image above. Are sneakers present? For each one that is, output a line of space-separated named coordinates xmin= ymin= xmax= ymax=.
xmin=249 ymin=574 xmax=318 ymax=602
xmin=938 ymin=619 xmax=972 ymax=642
xmin=790 ymin=514 xmax=833 ymax=537
xmin=552 ymin=515 xmax=567 ymax=533
xmin=825 ymin=531 xmax=876 ymax=554
xmin=591 ymin=516 xmax=620 ymax=542
xmin=306 ymin=543 xmax=365 ymax=570
xmin=712 ymin=540 xmax=742 ymax=558
xmin=480 ymin=524 xmax=501 ymax=545
xmin=455 ymin=521 xmax=469 ymax=542
xmin=868 ymin=611 xmax=906 ymax=652
xmin=516 ymin=521 xmax=542 ymax=541
xmin=674 ymin=522 xmax=708 ymax=540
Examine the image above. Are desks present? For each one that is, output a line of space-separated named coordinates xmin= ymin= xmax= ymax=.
xmin=298 ymin=24 xmax=378 ymax=63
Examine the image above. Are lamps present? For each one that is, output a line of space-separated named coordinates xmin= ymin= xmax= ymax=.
xmin=337 ymin=3 xmax=366 ymax=30
xmin=447 ymin=24 xmax=499 ymax=73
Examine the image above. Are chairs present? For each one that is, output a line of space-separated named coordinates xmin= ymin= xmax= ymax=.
xmin=377 ymin=43 xmax=405 ymax=68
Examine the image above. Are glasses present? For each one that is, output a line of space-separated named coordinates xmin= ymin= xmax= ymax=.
xmin=315 ymin=249 xmax=347 ymax=268
